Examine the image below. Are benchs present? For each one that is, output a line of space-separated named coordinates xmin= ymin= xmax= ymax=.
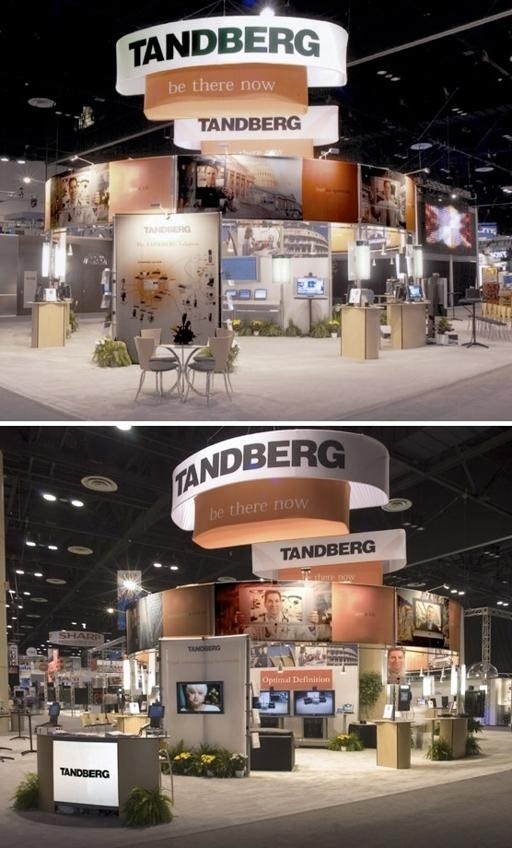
xmin=467 ymin=315 xmax=507 ymax=341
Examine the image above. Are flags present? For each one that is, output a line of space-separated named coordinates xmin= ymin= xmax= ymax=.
xmin=116 ymin=569 xmax=143 ymax=630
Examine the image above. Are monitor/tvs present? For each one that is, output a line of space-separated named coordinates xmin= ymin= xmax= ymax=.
xmin=255 ymin=691 xmax=290 ymax=717
xmin=174 ymin=680 xmax=224 ymax=714
xmin=148 ymin=706 xmax=166 ymax=717
xmin=431 ymin=698 xmax=436 ymax=707
xmin=48 ymin=705 xmax=60 ymax=716
xmin=465 ymin=690 xmax=485 ymax=717
xmin=294 ymin=689 xmax=336 ymax=718
xmin=441 ymin=696 xmax=448 ymax=708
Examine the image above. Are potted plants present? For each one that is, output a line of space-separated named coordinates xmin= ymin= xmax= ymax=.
xmin=232 ymin=319 xmax=341 ymax=337
xmin=69 ymin=309 xmax=79 ymax=332
xmin=168 ymin=312 xmax=198 ymax=345
xmin=435 ymin=317 xmax=455 ymax=345
xmin=159 ymin=738 xmax=249 ymax=778
xmin=92 ymin=335 xmax=132 ymax=367
xmin=328 ymin=733 xmax=363 ymax=752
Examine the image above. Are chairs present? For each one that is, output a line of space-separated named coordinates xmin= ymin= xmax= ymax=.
xmin=134 ymin=328 xmax=235 ymax=406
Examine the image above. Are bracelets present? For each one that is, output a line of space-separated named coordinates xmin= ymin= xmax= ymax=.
xmin=228 ymin=192 xmax=234 ymax=201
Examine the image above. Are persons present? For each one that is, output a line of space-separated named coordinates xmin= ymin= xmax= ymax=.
xmin=233 ymin=608 xmax=320 ymax=642
xmin=177 ymin=683 xmax=222 ymax=713
xmin=416 ymin=605 xmax=442 ymax=633
xmin=200 ymin=164 xmax=218 ymax=187
xmin=241 ymin=227 xmax=259 ymax=257
xmin=366 ymin=191 xmax=405 ymax=228
xmin=409 ymin=625 xmax=450 ymax=649
xmin=375 ymin=179 xmax=397 ymax=205
xmin=189 ymin=186 xmax=242 ymax=214
xmin=56 ymin=177 xmax=99 ymax=230
xmin=387 ymin=650 xmax=406 ymax=675
xmin=254 ymin=590 xmax=302 ymax=623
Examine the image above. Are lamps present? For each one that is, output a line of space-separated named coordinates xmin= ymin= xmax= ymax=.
xmin=277 ymin=641 xmax=283 ymax=672
xmin=340 ymin=647 xmax=345 ymax=673
xmin=439 ymin=655 xmax=446 ymax=683
xmin=419 ymin=653 xmax=424 ymax=677
xmin=67 ymin=226 xmax=73 ymax=256
